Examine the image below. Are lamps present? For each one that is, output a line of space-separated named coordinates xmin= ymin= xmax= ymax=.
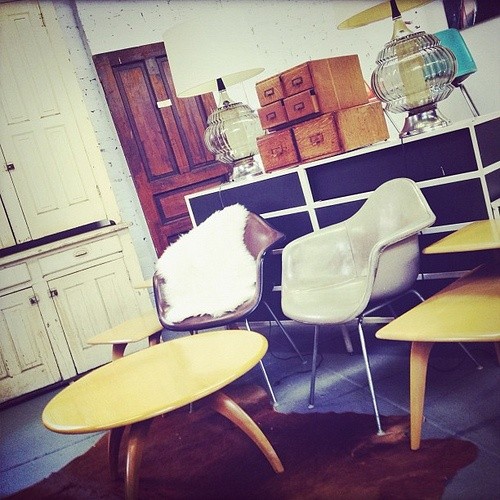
xmin=334 ymin=0 xmax=457 ymax=139
xmin=163 ymin=16 xmax=264 ymax=183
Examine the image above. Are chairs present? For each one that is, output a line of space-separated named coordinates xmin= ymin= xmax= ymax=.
xmin=154 ymin=203 xmax=307 ymax=410
xmin=281 ymin=178 xmax=484 ymax=437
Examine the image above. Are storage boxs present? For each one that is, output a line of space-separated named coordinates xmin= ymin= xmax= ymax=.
xmin=255 ymin=54 xmax=390 ymax=173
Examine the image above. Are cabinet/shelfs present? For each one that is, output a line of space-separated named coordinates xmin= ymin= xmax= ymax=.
xmin=0 ymin=0 xmax=165 ymax=411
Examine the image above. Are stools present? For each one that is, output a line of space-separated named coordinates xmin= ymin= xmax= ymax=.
xmin=42 ymin=330 xmax=284 ymax=500
xmin=83 ymin=309 xmax=164 ymax=361
xmin=375 ymin=262 xmax=500 ymax=450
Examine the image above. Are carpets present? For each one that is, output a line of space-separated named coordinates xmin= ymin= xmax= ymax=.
xmin=0 ymin=384 xmax=479 ymax=500
